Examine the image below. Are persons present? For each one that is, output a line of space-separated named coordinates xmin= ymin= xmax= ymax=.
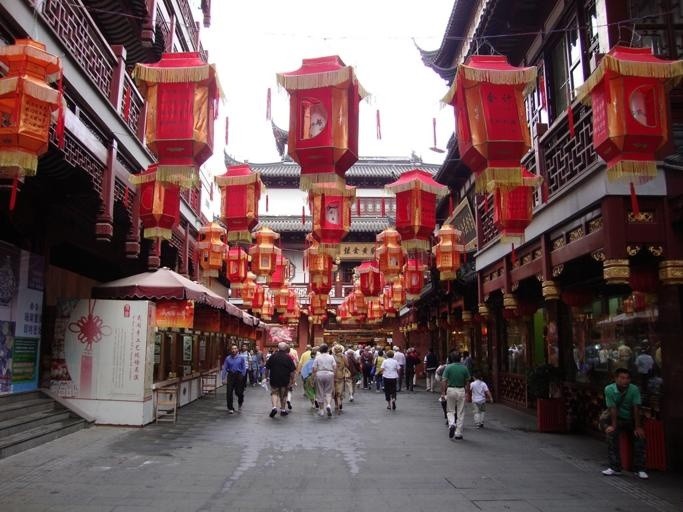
xmin=600 ymin=366 xmax=651 ymax=479
xmin=221 ymin=336 xmax=494 ymax=441
xmin=507 ymin=339 xmax=527 ymax=375
xmin=572 ymin=338 xmax=662 ymax=381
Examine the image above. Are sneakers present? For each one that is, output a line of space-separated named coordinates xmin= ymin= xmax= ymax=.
xmin=270 ymin=400 xmax=292 ymax=418
xmin=602 ymin=468 xmax=623 ymax=475
xmin=635 ymin=471 xmax=649 ymax=478
xmin=312 ymin=395 xmax=353 ymax=416
xmin=387 ymin=400 xmax=396 ymax=409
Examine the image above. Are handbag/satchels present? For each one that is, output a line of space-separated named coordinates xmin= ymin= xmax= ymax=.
xmin=598 ymin=408 xmax=610 ymax=431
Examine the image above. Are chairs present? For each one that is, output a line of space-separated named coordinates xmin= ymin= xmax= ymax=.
xmin=154 ymin=386 xmax=180 ymax=424
xmin=199 ymin=374 xmax=218 ymax=400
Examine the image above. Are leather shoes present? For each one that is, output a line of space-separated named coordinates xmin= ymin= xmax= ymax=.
xmin=449 ymin=425 xmax=463 ymax=440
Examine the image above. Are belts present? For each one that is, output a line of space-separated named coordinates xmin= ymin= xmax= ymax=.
xmin=448 ymin=385 xmax=464 ymax=387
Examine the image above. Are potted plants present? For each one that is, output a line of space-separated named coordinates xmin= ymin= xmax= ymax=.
xmin=527 ymin=362 xmax=567 ymax=434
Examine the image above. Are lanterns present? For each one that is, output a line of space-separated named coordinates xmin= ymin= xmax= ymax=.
xmin=568 ymin=47 xmax=683 ymax=217
xmin=0 ymin=37 xmax=65 ymax=197
xmin=266 ymin=55 xmax=382 ymax=227
xmin=124 ymin=51 xmax=225 ymax=185
xmin=438 ymin=55 xmax=537 ymax=220
xmin=197 ymin=223 xmax=467 ymax=329
xmin=129 ymin=161 xmax=543 ymax=262
